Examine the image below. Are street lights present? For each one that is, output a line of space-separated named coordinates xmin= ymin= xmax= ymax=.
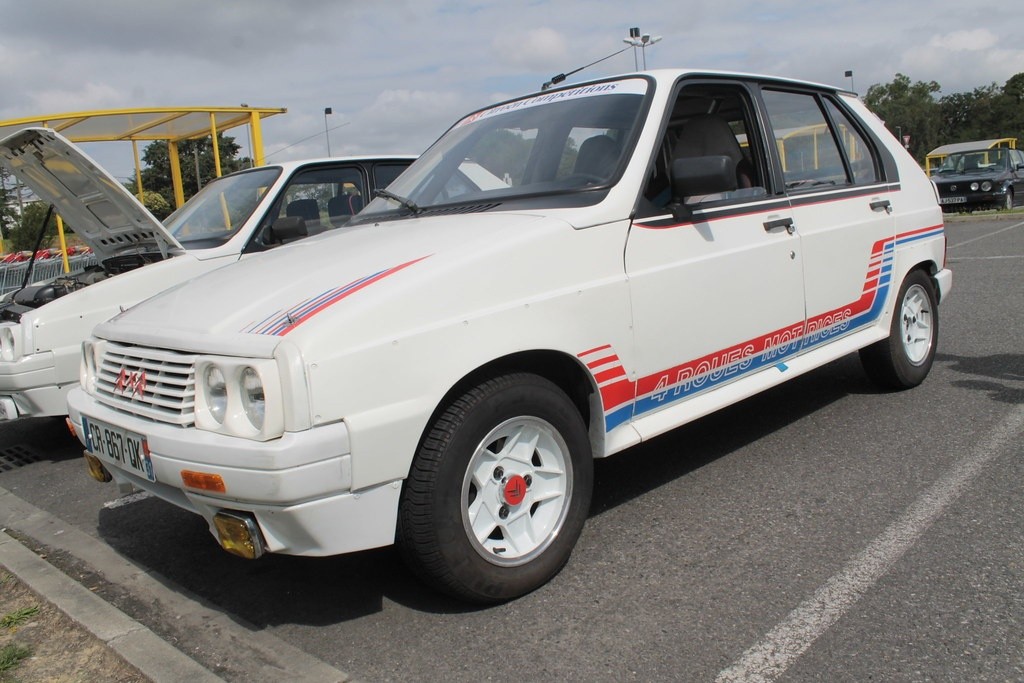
xmin=630 ymin=28 xmax=640 ymax=72
xmin=845 ymin=71 xmax=854 ymax=90
xmin=642 ymin=34 xmax=649 ymax=70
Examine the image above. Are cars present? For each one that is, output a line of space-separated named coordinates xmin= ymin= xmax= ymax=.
xmin=62 ymin=67 xmax=952 ymax=601
xmin=931 ymin=147 xmax=1024 ymax=211
xmin=0 ymin=127 xmax=512 ymax=423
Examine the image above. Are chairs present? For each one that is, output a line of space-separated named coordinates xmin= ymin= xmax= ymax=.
xmin=571 ymin=135 xmax=619 ymax=183
xmin=327 ymin=194 xmax=361 ymax=227
xmin=664 ymin=112 xmax=745 ymax=215
xmin=285 ymin=199 xmax=320 ymax=225
